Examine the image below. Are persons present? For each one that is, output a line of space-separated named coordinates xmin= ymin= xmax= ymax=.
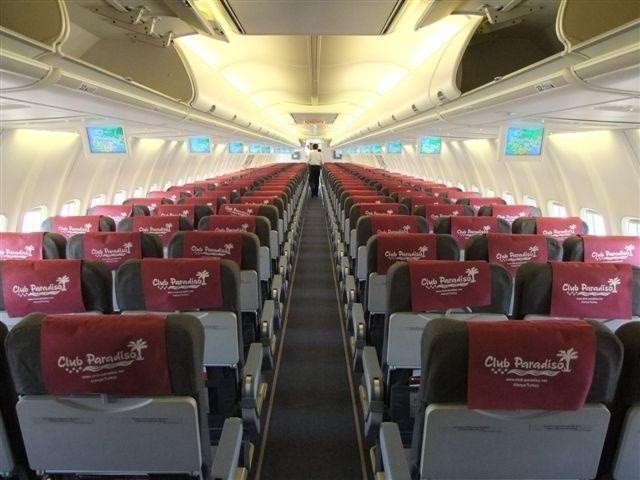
xmin=318 ymin=149 xmax=324 ymax=168
xmin=308 ymin=143 xmax=321 ymax=197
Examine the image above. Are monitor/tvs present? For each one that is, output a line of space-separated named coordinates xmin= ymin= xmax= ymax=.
xmin=249 ymin=144 xmax=260 ymax=154
xmin=83 ymin=124 xmax=129 ymax=156
xmin=274 ymin=145 xmax=300 ymax=159
xmin=189 ymin=136 xmax=210 ymax=154
xmin=262 ymin=145 xmax=270 ymax=153
xmin=503 ymin=125 xmax=547 ymax=160
xmin=229 ymin=142 xmax=243 ymax=153
xmin=372 ymin=144 xmax=382 ymax=154
xmin=385 ymin=142 xmax=403 ymax=155
xmin=417 ymin=137 xmax=442 ymax=155
xmin=334 ymin=145 xmax=368 ymax=159
